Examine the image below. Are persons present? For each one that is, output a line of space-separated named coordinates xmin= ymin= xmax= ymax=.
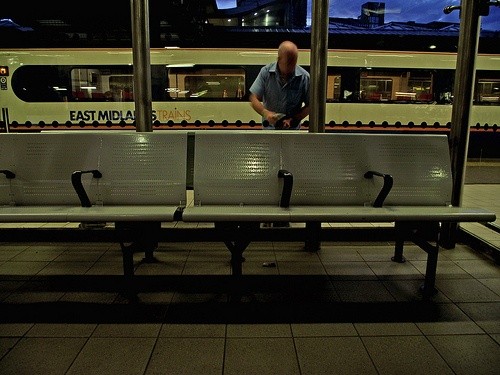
xmin=248 ymin=40 xmax=310 ymax=130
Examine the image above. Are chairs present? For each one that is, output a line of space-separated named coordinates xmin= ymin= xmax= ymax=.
xmin=72 ymin=90 xmax=135 ymax=102
xmin=359 ymin=84 xmax=433 ymax=102
xmin=169 ymin=87 xmax=244 ymax=100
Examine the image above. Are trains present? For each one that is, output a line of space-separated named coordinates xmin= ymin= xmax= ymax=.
xmin=0 ymin=45 xmax=500 ymax=154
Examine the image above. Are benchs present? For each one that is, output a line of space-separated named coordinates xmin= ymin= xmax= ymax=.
xmin=1 ymin=132 xmax=191 ymax=294
xmin=176 ymin=130 xmax=498 ymax=297
